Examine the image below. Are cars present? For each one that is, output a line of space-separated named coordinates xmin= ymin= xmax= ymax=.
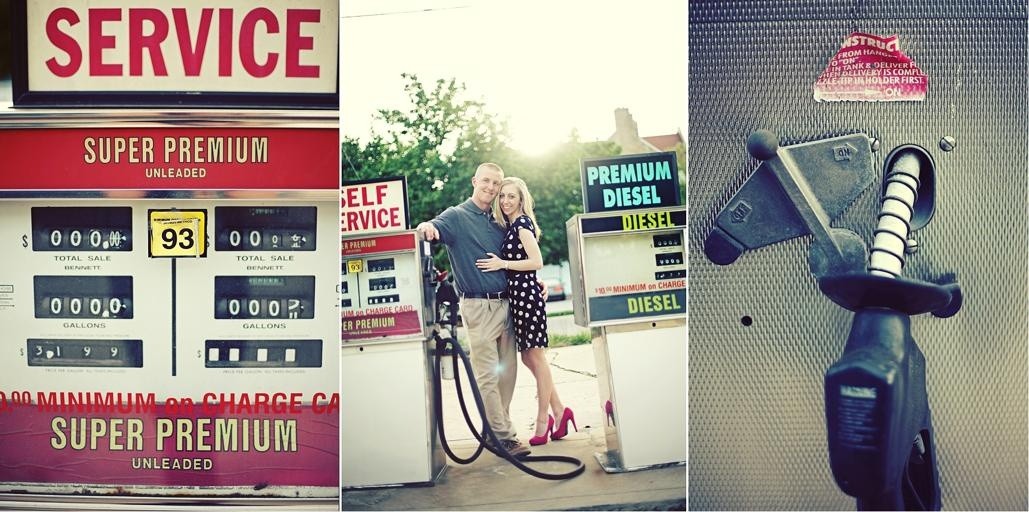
xmin=543 ymin=278 xmax=566 ymax=301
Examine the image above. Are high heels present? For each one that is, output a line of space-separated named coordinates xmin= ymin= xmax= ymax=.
xmin=528 ymin=414 xmax=555 ymax=446
xmin=550 ymin=407 xmax=578 ymax=440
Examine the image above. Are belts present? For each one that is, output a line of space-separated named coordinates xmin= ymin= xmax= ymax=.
xmin=458 ymin=291 xmax=509 ymax=299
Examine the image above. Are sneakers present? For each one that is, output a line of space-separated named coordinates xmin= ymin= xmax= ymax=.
xmin=500 ymin=438 xmax=531 ymax=456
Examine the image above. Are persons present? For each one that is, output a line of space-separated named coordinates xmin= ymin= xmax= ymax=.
xmin=417 ymin=162 xmax=577 ymax=456
